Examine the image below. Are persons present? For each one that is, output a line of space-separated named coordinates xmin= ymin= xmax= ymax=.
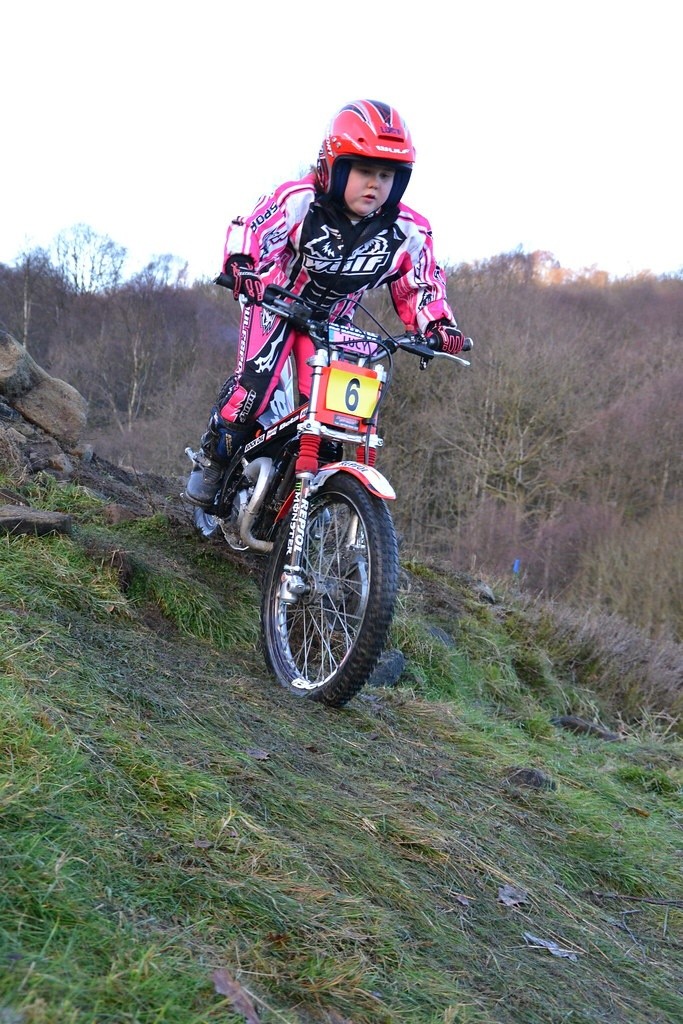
xmin=183 ymin=99 xmax=464 ymax=508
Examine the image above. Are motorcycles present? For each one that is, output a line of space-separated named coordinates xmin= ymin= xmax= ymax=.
xmin=184 ymin=270 xmax=477 ymax=710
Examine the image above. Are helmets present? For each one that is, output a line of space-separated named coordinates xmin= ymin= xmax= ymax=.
xmin=317 ymin=100 xmax=416 ymax=206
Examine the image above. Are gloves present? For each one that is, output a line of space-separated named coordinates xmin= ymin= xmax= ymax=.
xmin=424 ymin=318 xmax=465 ymax=355
xmin=224 ymin=253 xmax=265 ymax=307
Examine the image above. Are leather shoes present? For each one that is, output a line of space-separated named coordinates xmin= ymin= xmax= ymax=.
xmin=185 ymin=458 xmax=228 ymax=508
